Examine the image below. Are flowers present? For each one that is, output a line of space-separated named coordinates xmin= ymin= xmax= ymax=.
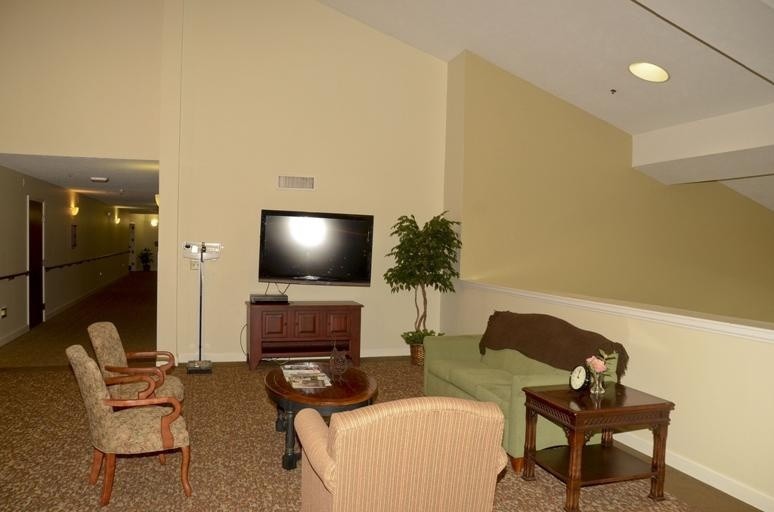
xmin=585 ymin=349 xmax=614 ymax=379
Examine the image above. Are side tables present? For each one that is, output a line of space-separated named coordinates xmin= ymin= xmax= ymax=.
xmin=521 ymin=380 xmax=674 ymax=512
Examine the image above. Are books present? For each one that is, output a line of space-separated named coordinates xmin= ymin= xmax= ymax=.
xmin=279 ymin=363 xmax=332 ymax=390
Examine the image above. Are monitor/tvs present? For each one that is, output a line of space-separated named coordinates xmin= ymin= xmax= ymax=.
xmin=258 ymin=209 xmax=374 ymax=287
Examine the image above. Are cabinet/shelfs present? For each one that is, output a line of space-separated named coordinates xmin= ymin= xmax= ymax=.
xmin=245 ymin=301 xmax=364 ymax=371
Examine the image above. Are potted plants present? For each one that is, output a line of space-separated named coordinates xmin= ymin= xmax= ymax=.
xmin=384 ymin=209 xmax=464 ymax=366
xmin=138 ymin=247 xmax=154 ymax=272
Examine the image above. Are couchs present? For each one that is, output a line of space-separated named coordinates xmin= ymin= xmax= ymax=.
xmin=423 ymin=311 xmax=629 ymax=479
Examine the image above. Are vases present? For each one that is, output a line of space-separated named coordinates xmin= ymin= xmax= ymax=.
xmin=590 ymin=375 xmax=606 ymax=394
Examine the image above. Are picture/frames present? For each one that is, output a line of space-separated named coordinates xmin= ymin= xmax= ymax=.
xmin=69 ymin=222 xmax=79 ymax=249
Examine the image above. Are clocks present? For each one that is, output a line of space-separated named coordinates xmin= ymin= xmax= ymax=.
xmin=568 ymin=365 xmax=590 ymax=392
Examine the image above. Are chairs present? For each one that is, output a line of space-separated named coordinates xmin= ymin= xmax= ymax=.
xmin=293 ymin=396 xmax=509 ymax=512
xmin=87 ymin=321 xmax=185 ymax=464
xmin=65 ymin=344 xmax=193 ymax=507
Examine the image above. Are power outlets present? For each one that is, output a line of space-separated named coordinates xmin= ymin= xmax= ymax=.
xmin=200 ymin=241 xmax=220 ymax=259
